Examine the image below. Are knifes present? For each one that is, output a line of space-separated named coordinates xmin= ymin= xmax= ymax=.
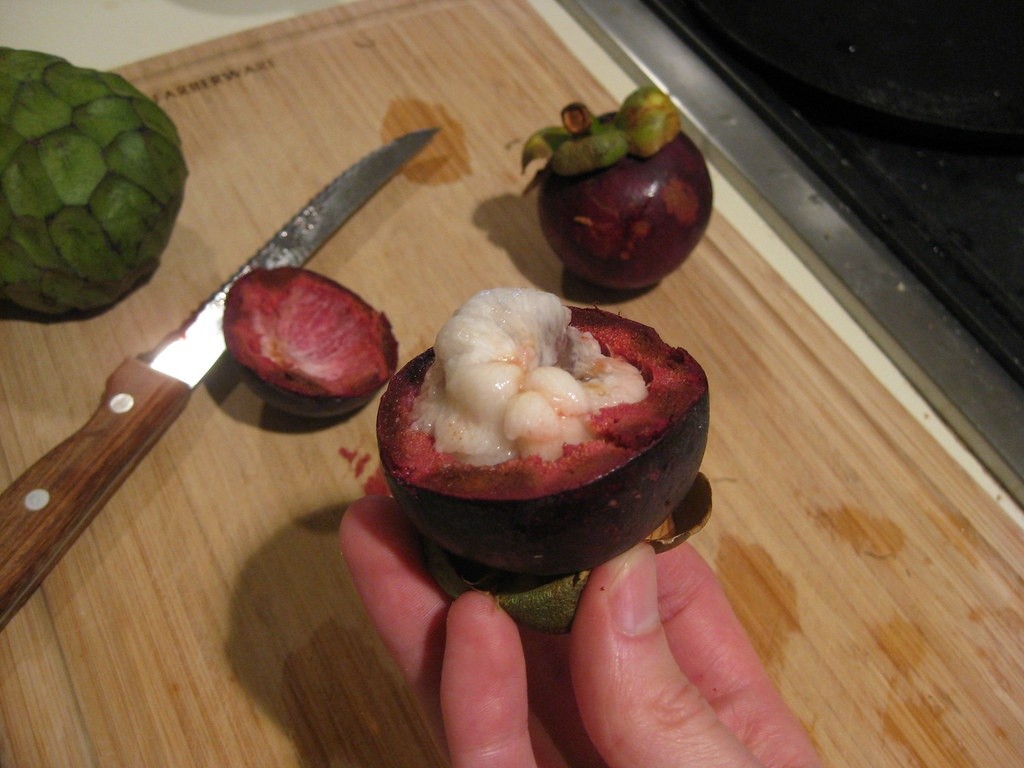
xmin=0 ymin=128 xmax=442 ymax=632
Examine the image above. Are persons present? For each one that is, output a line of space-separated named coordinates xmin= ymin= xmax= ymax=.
xmin=337 ymin=487 xmax=831 ymax=768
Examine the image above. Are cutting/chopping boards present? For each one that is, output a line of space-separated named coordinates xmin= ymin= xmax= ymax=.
xmin=0 ymin=0 xmax=1024 ymax=768
